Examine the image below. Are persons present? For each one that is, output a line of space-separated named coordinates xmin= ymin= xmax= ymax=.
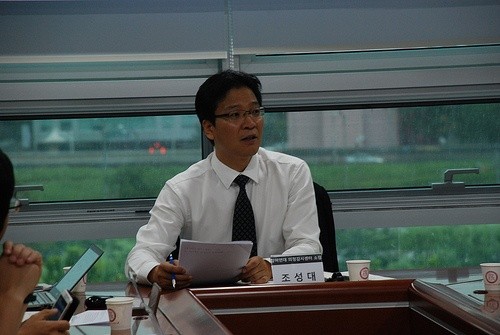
xmin=124 ymin=69 xmax=324 ymax=290
xmin=0 ymin=149 xmax=73 ymax=335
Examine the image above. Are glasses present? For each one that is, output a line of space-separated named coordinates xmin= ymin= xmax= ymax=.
xmin=212 ymin=106 xmax=265 ymax=122
xmin=8 ymin=197 xmax=23 ymax=215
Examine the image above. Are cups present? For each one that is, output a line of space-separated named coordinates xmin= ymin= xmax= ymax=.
xmin=345 ymin=259 xmax=372 ymax=281
xmin=62 ymin=266 xmax=88 ymax=295
xmin=105 ymin=297 xmax=134 ymax=330
xmin=479 ymin=264 xmax=500 ymax=291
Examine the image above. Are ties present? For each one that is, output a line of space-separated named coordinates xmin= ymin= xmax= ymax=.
xmin=231 ymin=174 xmax=258 ymax=261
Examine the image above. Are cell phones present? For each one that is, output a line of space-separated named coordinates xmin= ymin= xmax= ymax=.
xmin=48 ymin=290 xmax=80 ymax=321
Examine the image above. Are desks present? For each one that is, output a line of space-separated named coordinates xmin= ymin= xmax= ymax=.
xmin=63 ymin=266 xmax=500 ymax=335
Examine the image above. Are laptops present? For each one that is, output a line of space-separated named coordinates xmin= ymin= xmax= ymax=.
xmin=26 ymin=244 xmax=104 ymax=311
